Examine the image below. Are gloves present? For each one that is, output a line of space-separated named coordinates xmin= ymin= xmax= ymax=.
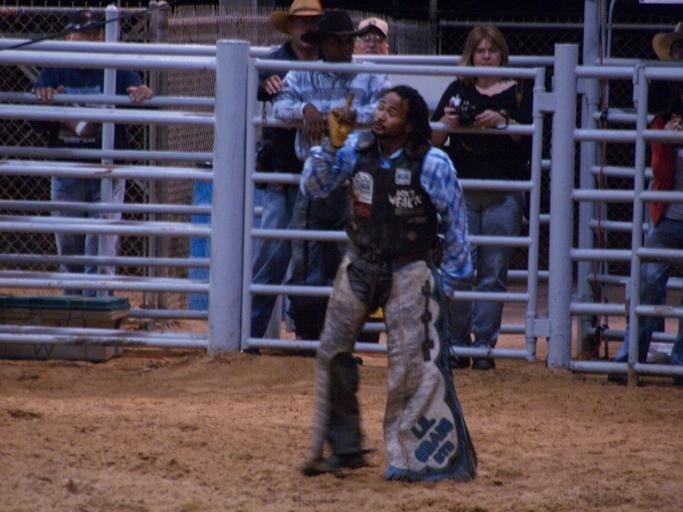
xmin=327 ymin=92 xmax=357 ymax=148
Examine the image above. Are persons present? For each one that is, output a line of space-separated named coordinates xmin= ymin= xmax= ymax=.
xmin=270 ymin=10 xmax=391 ymax=365
xmin=353 ymin=16 xmax=390 ymax=56
xmin=427 ymin=24 xmax=533 ymax=373
xmin=298 ymin=84 xmax=480 ymax=482
xmin=30 ymin=9 xmax=156 ymax=296
xmin=605 ymin=23 xmax=682 ymax=388
xmin=241 ymin=1 xmax=331 ymax=354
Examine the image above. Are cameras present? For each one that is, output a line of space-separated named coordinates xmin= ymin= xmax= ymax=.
xmin=451 ymin=94 xmax=480 ymax=128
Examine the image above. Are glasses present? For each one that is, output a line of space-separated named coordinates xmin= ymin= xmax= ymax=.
xmin=360 ymin=34 xmax=384 ymax=42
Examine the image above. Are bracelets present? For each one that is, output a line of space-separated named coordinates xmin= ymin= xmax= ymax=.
xmin=496 ymin=110 xmax=509 ymax=130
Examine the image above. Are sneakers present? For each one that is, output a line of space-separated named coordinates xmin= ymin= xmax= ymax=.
xmin=320 ymin=454 xmax=364 ymax=468
xmin=451 ymin=342 xmax=496 ymax=369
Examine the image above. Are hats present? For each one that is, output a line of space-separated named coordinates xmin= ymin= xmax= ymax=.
xmin=271 ymin=0 xmax=323 ymax=32
xmin=355 ymin=17 xmax=389 ymax=39
xmin=66 ymin=10 xmax=103 ymax=32
xmin=652 ymin=22 xmax=682 ymax=60
xmin=301 ymin=11 xmax=370 ymax=44
